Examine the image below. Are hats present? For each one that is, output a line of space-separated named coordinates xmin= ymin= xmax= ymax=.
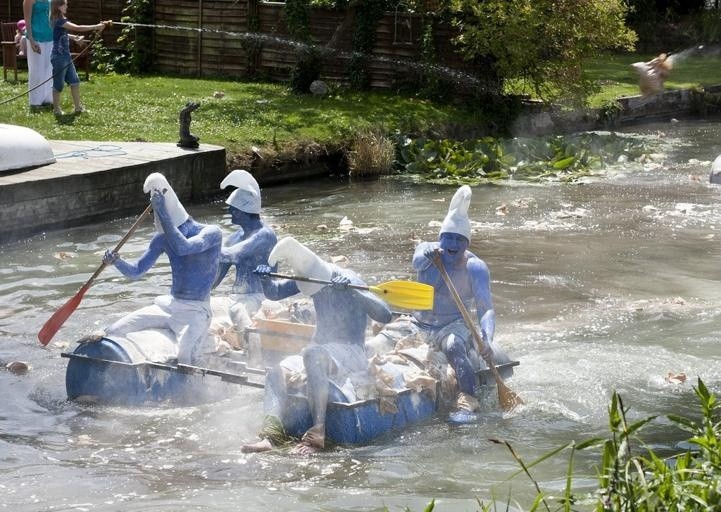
xmin=17 ymin=20 xmax=26 ymax=30
xmin=268 ymin=237 xmax=332 ymax=296
xmin=143 ymin=172 xmax=189 ymax=231
xmin=220 ymin=170 xmax=261 ymax=214
xmin=439 ymin=185 xmax=472 ymax=246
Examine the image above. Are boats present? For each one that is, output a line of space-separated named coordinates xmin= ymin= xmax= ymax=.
xmin=61 ymin=293 xmax=520 ymax=449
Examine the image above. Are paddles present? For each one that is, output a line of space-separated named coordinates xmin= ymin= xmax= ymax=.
xmin=37 ymin=188 xmax=168 ymax=347
xmin=431 ymin=254 xmax=524 ymax=411
xmin=252 ymin=270 xmax=435 ymax=311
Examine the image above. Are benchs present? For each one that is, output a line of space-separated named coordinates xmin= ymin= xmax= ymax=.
xmin=1 ymin=22 xmax=90 ymax=81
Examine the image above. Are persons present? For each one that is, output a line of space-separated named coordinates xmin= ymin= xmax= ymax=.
xmin=104 ymin=189 xmax=225 ymax=386
xmin=14 ymin=19 xmax=28 ymax=56
xmin=24 ymin=0 xmax=56 ymax=114
xmin=49 ymin=1 xmax=115 ymax=117
xmin=204 ymin=204 xmax=279 ymax=350
xmin=237 ymin=263 xmax=392 ymax=457
xmin=373 ymin=233 xmax=498 ymax=427
